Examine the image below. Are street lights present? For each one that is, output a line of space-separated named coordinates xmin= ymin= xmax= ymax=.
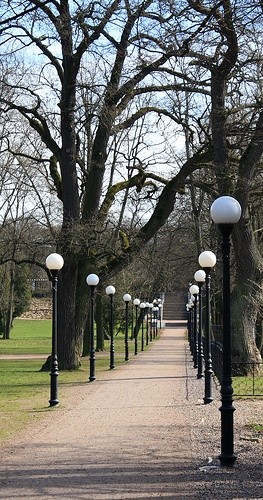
xmin=87 ymin=272 xmax=100 ymax=381
xmin=145 ymin=298 xmax=162 ymax=345
xmin=197 ymin=249 xmax=217 ymax=404
xmin=139 ymin=301 xmax=145 ymax=351
xmin=122 ymin=293 xmax=131 ymax=361
xmin=105 ymin=284 xmax=116 ymax=368
xmin=194 ymin=269 xmax=206 ymax=379
xmin=208 ymin=195 xmax=243 ymax=468
xmin=45 ymin=252 xmax=65 ymax=407
xmin=185 ymin=284 xmax=199 ymax=368
xmin=133 ymin=298 xmax=140 ymax=355
